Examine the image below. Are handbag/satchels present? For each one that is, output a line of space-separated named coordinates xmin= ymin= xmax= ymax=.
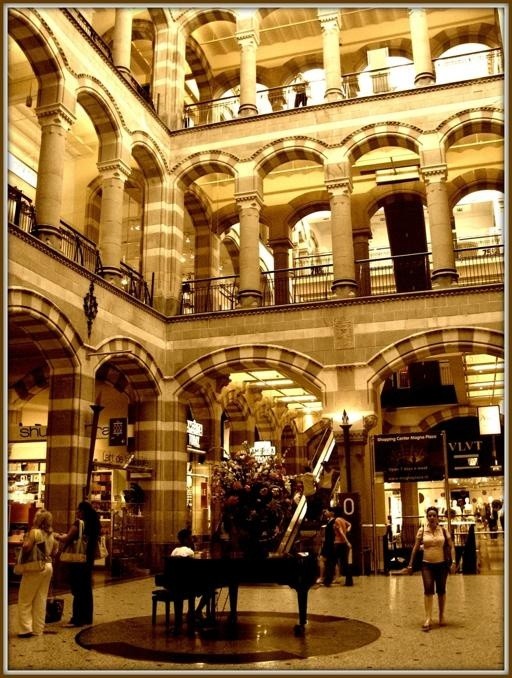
xmin=58 ymin=534 xmax=89 ymax=565
xmin=411 ymin=544 xmax=423 ymax=572
xmin=12 ymin=540 xmax=47 ymax=576
xmin=442 ymin=543 xmax=460 ymax=575
xmin=93 ymin=535 xmax=110 ymax=561
xmin=44 ymin=598 xmax=64 ymax=623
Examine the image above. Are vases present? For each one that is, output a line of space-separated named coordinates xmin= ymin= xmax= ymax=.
xmin=241 ymin=540 xmax=270 ymax=561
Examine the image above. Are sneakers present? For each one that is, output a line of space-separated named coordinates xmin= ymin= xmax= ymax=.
xmin=422 ymin=618 xmax=446 ymax=631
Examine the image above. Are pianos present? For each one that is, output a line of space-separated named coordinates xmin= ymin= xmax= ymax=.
xmin=162 ymin=548 xmax=319 ymax=638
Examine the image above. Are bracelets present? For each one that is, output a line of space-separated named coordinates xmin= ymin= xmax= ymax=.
xmin=452 ymin=559 xmax=456 ymax=563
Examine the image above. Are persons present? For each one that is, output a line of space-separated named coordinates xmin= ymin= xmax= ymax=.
xmin=53 ymin=499 xmax=104 ymax=628
xmin=319 ymin=506 xmax=355 ymax=587
xmin=16 ymin=507 xmax=58 ymax=638
xmin=165 ymin=527 xmax=212 ymax=621
xmin=451 ymin=498 xmax=462 ymax=520
xmin=485 ymin=503 xmax=500 ymax=545
xmin=463 ymin=496 xmax=475 ymax=519
xmin=319 ymin=506 xmax=338 ymax=584
xmin=292 ymin=71 xmax=311 ymax=108
xmin=406 ymin=505 xmax=456 ymax=630
xmin=299 ymin=465 xmax=320 ymax=523
xmin=318 ymin=460 xmax=339 ymax=507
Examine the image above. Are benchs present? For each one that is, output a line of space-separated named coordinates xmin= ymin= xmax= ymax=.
xmin=150 ymin=585 xmax=220 ymax=628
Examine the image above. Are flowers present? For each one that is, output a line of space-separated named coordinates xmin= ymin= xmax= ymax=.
xmin=191 ymin=437 xmax=306 ymax=542
